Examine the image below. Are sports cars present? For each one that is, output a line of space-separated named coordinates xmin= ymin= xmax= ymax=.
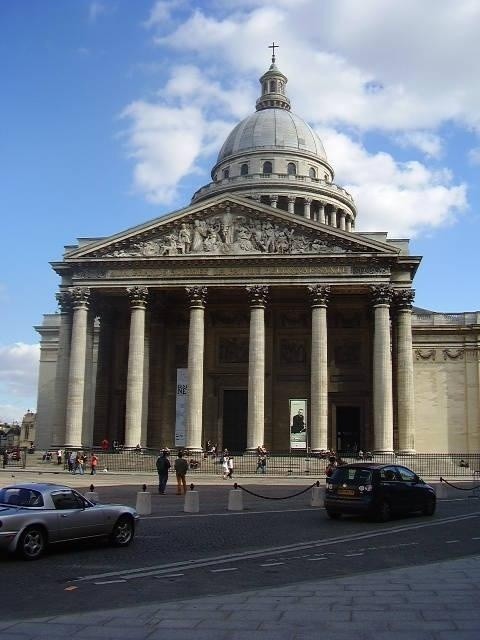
xmin=0 ymin=483 xmax=140 ymax=561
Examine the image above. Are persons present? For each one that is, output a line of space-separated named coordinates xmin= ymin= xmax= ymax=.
xmin=54 ymin=498 xmax=66 ymax=509
xmin=460 ymin=460 xmax=469 ymax=467
xmin=156 ymin=440 xmax=234 ymax=495
xmin=384 ymin=471 xmax=400 ymax=480
xmin=319 ymin=442 xmax=374 ymax=476
xmin=2 ymin=437 xmax=145 ymax=476
xmin=293 ymin=408 xmax=306 ymax=433
xmin=100 ymin=206 xmax=364 ymax=258
xmin=18 ymin=490 xmax=41 ymax=505
xmin=254 ymin=444 xmax=268 ymax=474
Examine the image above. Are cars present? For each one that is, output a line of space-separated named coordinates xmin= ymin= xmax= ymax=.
xmin=323 ymin=462 xmax=436 ymax=523
